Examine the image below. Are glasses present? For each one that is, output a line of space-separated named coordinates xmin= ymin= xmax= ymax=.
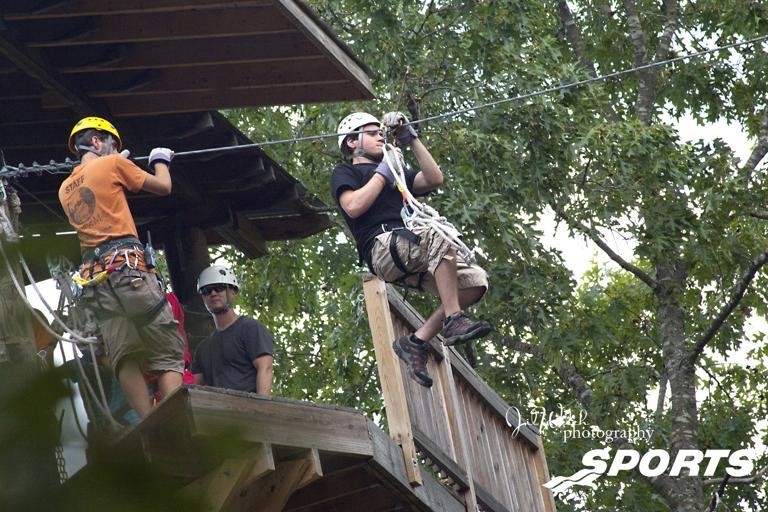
xmin=200 ymin=286 xmax=227 ymax=294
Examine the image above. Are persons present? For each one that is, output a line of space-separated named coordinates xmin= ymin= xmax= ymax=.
xmin=332 ymin=111 xmax=491 ymax=387
xmin=57 ymin=117 xmax=195 ymax=448
xmin=193 ymin=266 xmax=273 ymax=395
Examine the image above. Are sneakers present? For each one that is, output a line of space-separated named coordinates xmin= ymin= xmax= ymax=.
xmin=443 ymin=310 xmax=491 ymax=346
xmin=392 ymin=333 xmax=432 ymax=387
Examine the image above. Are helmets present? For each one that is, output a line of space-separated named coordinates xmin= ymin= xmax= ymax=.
xmin=68 ymin=116 xmax=122 ymax=159
xmin=196 ymin=265 xmax=239 ymax=294
xmin=337 ymin=112 xmax=381 ymax=156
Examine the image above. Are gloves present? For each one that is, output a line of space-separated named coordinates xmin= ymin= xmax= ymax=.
xmin=148 ymin=148 xmax=174 ymax=167
xmin=373 ymin=111 xmax=418 ymax=188
